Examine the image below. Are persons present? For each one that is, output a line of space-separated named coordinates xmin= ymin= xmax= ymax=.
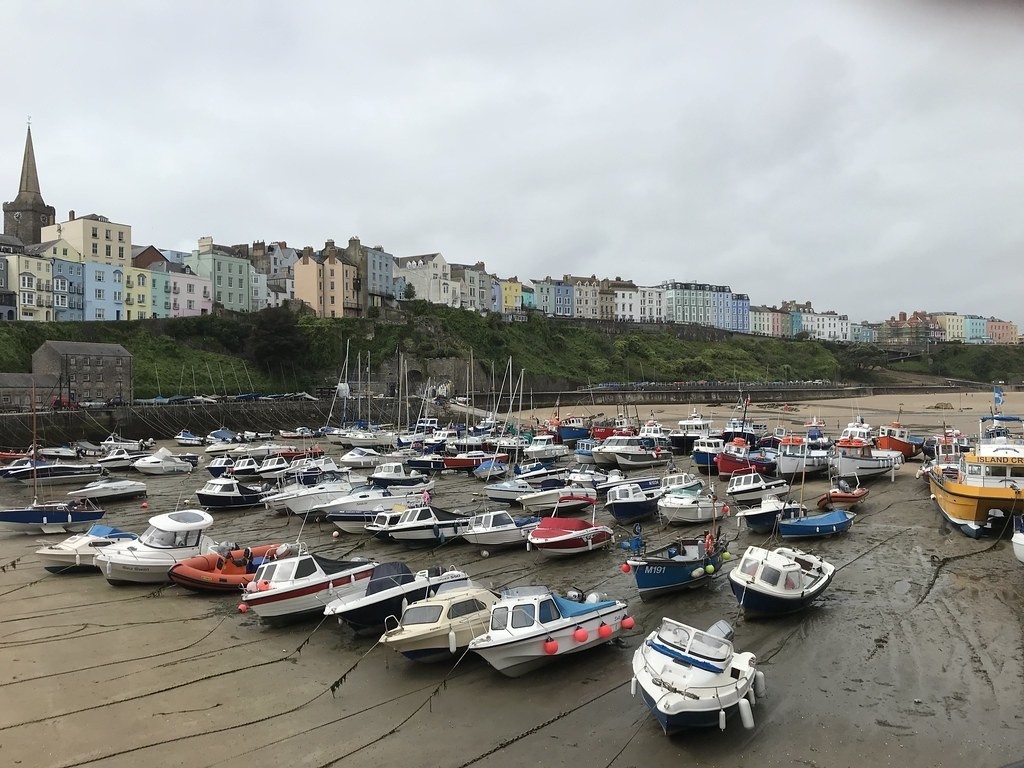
xmin=421 ymin=488 xmax=431 ymax=505
xmin=667 ymin=460 xmax=676 ymax=474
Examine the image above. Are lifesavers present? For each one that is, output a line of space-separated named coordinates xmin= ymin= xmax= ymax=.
xmin=735 ymin=437 xmax=745 ymax=446
xmin=782 ymin=436 xmax=803 ymax=444
xmin=705 ymin=533 xmax=714 ymax=555
xmin=839 ymin=437 xmax=851 ymax=446
xmin=852 ymin=439 xmax=863 ymax=447
xmin=891 ymin=422 xmax=900 ymax=429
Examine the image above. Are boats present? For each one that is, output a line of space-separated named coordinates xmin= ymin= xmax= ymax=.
xmin=0 ymin=461 xmax=108 ymax=487
xmin=97 ymin=447 xmax=155 ymax=473
xmin=527 ymin=495 xmax=617 ymax=557
xmin=129 ymin=446 xmax=194 ymax=476
xmin=92 ymin=508 xmax=217 ymax=588
xmin=460 ymin=508 xmax=542 ymax=549
xmin=137 ymin=338 xmax=1024 ymax=563
xmin=99 ymin=430 xmax=156 ymax=453
xmin=377 ymin=580 xmax=534 ymax=668
xmin=26 ymin=442 xmax=86 ymax=461
xmin=65 ymin=478 xmax=147 ymax=505
xmin=0 ymin=447 xmax=49 ymax=476
xmin=727 ymin=543 xmax=836 ymax=627
xmin=166 ymin=499 xmax=314 ymax=595
xmin=626 ymin=482 xmax=730 ymax=602
xmin=323 ymin=561 xmax=470 ymax=638
xmin=35 ymin=522 xmax=141 ymax=575
xmin=238 ymin=542 xmax=380 ymax=630
xmin=630 ymin=619 xmax=759 ymax=737
xmin=469 ymin=584 xmax=630 ymax=680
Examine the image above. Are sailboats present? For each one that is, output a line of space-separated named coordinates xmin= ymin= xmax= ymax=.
xmin=0 ymin=375 xmax=107 ymax=535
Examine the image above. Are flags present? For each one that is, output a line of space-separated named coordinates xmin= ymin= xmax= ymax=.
xmin=994 ymin=387 xmax=1004 ymax=405
xmin=745 ymin=393 xmax=751 ymax=406
xmin=556 ymin=397 xmax=560 ymax=406
